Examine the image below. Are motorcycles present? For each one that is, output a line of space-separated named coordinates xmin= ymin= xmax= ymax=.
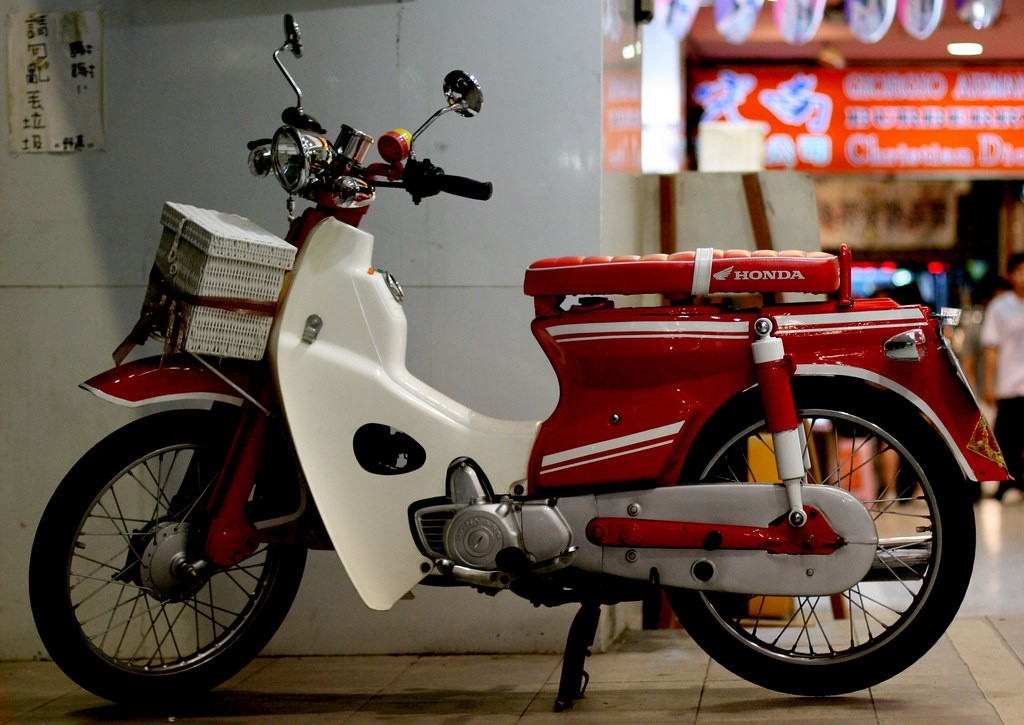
xmin=27 ymin=13 xmax=1016 ymax=714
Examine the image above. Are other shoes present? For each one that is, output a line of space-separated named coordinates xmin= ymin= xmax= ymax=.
xmin=1001 ymin=486 xmax=1022 ymax=507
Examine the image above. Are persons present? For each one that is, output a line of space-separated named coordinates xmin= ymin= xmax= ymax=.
xmin=980 ymin=252 xmax=1024 ymax=503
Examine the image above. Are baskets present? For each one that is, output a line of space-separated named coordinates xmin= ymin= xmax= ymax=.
xmin=139 ymin=199 xmax=299 ymax=363
